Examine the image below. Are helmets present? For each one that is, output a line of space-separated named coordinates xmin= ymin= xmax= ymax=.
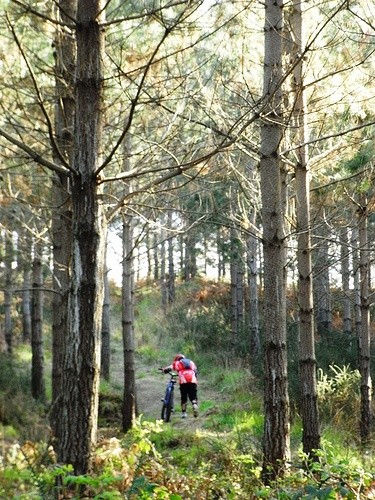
xmin=174 ymin=354 xmax=185 ymax=361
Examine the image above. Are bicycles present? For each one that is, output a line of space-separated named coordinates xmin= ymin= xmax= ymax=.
xmin=158 ymin=367 xmax=180 ymax=423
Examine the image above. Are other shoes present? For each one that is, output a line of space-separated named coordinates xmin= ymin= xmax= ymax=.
xmin=193 ymin=406 xmax=198 ymax=417
xmin=181 ymin=412 xmax=187 ymax=418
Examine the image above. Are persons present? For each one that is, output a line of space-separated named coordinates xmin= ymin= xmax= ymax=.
xmin=163 ymin=354 xmax=199 ymax=418
